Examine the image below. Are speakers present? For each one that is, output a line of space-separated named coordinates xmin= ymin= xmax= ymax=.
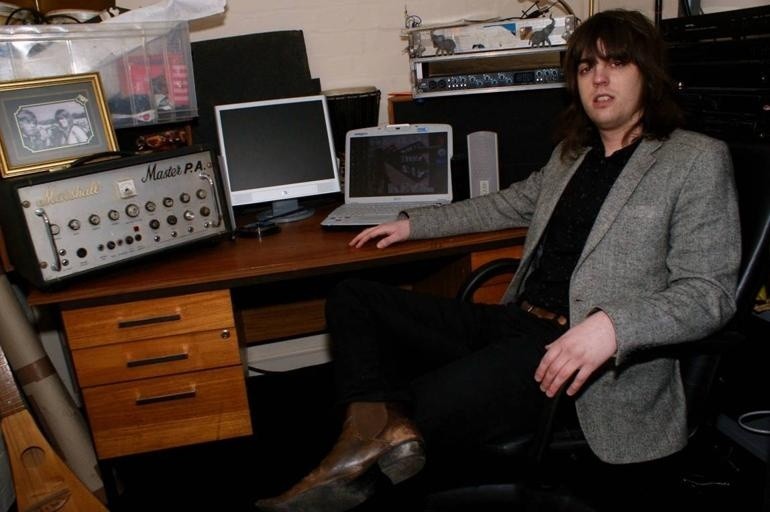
xmin=466 ymin=131 xmax=500 ymax=199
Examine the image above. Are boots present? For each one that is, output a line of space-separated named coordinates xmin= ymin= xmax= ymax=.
xmin=253 ymin=401 xmax=426 ymax=511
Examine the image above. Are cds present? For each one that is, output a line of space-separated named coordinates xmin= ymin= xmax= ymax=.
xmin=239 ymin=221 xmax=275 ymax=233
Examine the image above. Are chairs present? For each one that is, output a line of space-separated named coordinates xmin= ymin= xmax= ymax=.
xmin=432 ymin=146 xmax=768 ymax=511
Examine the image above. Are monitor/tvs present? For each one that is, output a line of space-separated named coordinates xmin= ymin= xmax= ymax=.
xmin=190 ymin=30 xmax=315 ymax=143
xmin=214 ymin=94 xmax=342 ymax=224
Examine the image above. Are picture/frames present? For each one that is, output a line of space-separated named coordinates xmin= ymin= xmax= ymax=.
xmin=1 ymin=71 xmax=122 ymax=181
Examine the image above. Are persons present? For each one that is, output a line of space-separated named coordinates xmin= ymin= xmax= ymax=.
xmin=17 ymin=109 xmax=89 ymax=151
xmin=254 ymin=8 xmax=742 ymax=512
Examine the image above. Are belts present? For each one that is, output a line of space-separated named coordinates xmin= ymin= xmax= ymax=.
xmin=520 ymin=299 xmax=568 ymax=325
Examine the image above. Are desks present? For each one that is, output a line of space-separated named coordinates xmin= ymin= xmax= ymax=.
xmin=28 ymin=203 xmax=527 ymax=511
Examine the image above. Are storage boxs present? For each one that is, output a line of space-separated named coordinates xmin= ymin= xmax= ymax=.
xmin=0 ymin=19 xmax=199 ymax=131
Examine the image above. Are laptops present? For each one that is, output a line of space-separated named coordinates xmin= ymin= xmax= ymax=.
xmin=319 ymin=123 xmax=454 ymax=232
